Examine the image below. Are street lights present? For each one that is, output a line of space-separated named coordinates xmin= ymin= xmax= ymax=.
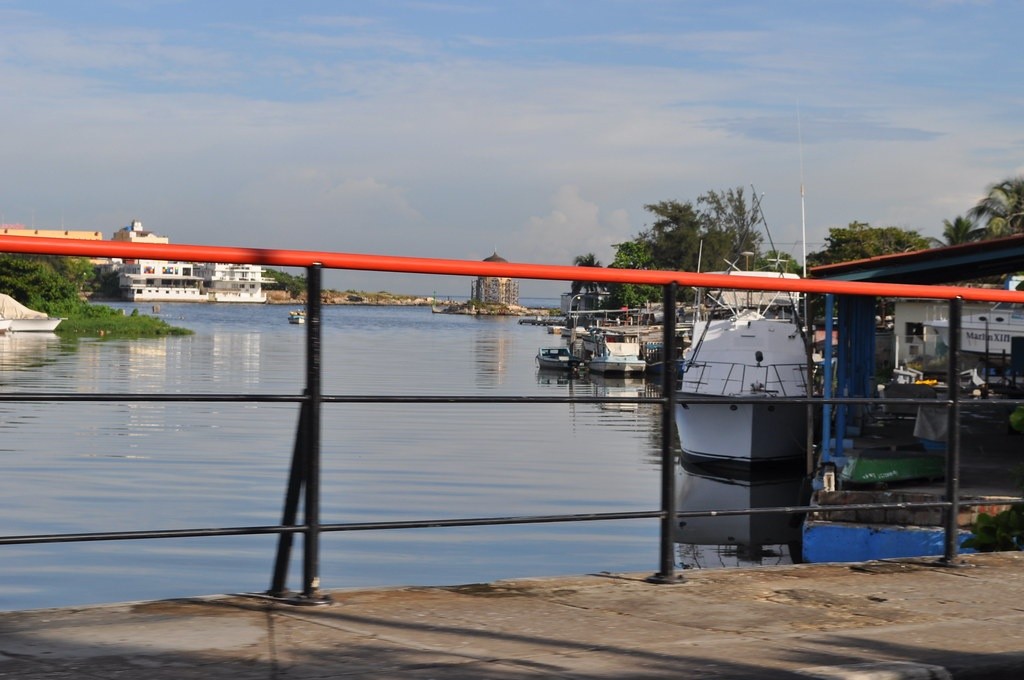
xmin=569 ymin=294 xmax=584 ymax=319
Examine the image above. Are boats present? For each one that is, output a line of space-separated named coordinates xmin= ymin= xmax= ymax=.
xmin=674 ymin=252 xmax=824 ymax=464
xmin=640 ymin=343 xmax=685 ymax=389
xmin=923 ymin=275 xmax=1024 ymax=354
xmin=536 ymin=346 xmax=583 ymax=370
xmin=287 ymin=309 xmax=307 ymax=323
xmin=587 ymin=342 xmax=646 ymax=371
